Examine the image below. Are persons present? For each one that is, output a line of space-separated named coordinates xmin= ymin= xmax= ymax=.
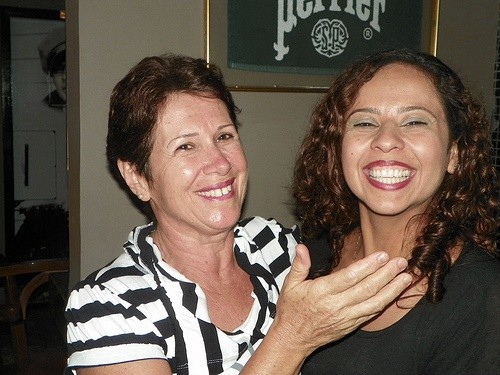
xmin=38 ymin=28 xmax=67 ymax=109
xmin=63 ymin=54 xmax=412 ymax=375
xmin=292 ymin=46 xmax=499 ymax=375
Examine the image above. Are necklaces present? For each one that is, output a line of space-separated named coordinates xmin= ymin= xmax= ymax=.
xmin=349 ymin=232 xmax=367 ymax=267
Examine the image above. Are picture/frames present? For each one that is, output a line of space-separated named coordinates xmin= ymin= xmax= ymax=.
xmin=205 ymin=0 xmax=441 ymax=94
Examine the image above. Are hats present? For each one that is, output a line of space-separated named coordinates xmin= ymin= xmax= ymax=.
xmin=39 ymin=24 xmax=70 ymax=75
